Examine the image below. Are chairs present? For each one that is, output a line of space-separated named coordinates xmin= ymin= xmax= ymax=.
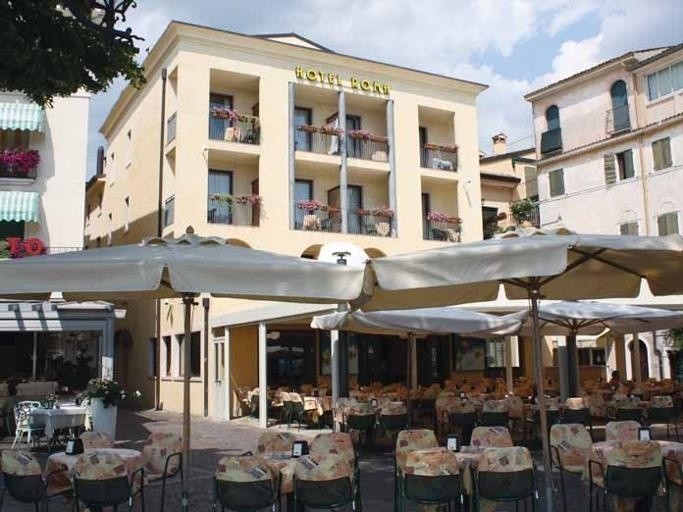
xmin=214 ymin=377 xmax=683 ymax=512
xmin=0 ymin=394 xmax=182 ymax=512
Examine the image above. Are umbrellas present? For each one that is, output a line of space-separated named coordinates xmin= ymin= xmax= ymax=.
xmin=0 ymin=224 xmax=364 ymax=511
xmin=508 ymin=300 xmax=683 ymax=396
xmin=362 ymin=221 xmax=683 ymax=512
xmin=309 ymin=308 xmax=522 ymax=431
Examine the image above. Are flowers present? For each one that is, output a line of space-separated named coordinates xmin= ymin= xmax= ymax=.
xmin=0 ymin=236 xmax=46 ymax=263
xmin=208 ymin=189 xmax=461 ymax=231
xmin=213 ymin=105 xmax=458 ymax=154
xmin=0 ymin=146 xmax=37 ymax=176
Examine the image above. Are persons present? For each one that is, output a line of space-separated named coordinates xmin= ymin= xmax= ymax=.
xmin=610 ymin=370 xmax=620 ymax=383
